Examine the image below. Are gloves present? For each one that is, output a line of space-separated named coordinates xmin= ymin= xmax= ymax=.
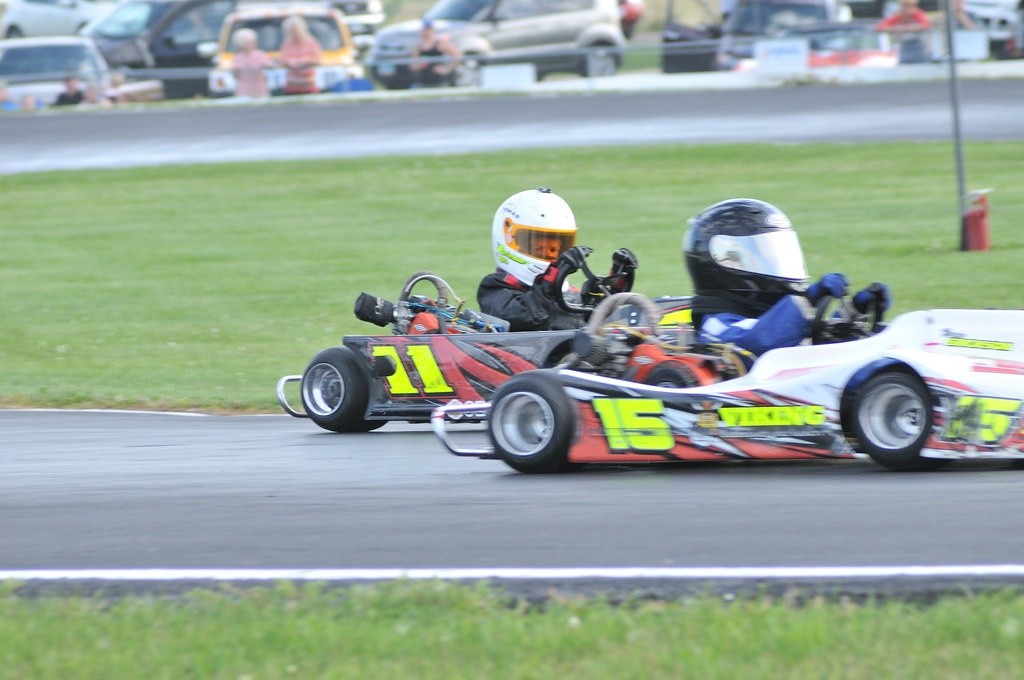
xmin=611 ymin=247 xmax=638 ymax=291
xmin=853 ymin=283 xmax=889 ymax=321
xmin=808 ymin=273 xmax=848 ymax=304
xmin=541 ymin=246 xmax=585 ymax=295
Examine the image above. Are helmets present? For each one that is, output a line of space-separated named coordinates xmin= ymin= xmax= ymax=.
xmin=682 ymin=199 xmax=811 ymax=296
xmin=493 ymin=187 xmax=578 ymax=287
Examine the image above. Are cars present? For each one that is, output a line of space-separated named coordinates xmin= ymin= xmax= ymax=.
xmin=1 ymin=0 xmax=123 ymax=39
xmin=957 ymin=0 xmax=1019 ymax=29
xmin=75 ymin=0 xmax=240 ymax=100
xmin=734 ymin=21 xmax=903 ymax=72
xmin=661 ymin=0 xmax=851 ymax=73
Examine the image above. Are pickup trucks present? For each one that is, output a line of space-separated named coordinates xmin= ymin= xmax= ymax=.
xmin=196 ymin=6 xmax=373 ymax=97
xmin=1 ymin=36 xmax=164 ymax=107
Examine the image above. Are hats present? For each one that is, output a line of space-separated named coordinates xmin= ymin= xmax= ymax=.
xmin=422 ymin=19 xmax=432 ymax=29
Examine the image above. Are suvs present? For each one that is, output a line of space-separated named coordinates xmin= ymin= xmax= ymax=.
xmin=362 ymin=1 xmax=628 ymax=90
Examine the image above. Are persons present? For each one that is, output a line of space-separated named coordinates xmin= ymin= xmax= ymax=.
xmin=681 ymin=197 xmax=890 ymax=378
xmin=0 ymin=15 xmax=459 ymax=109
xmin=476 ymin=185 xmax=640 ymax=330
xmin=874 ymin=0 xmax=982 ymax=64
